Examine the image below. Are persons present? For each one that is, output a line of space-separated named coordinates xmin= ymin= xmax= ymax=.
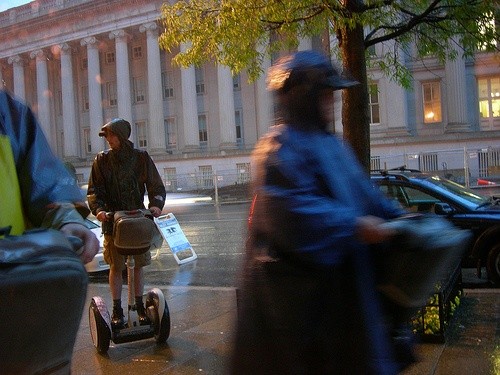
xmin=232 ymin=49 xmax=424 ymax=375
xmin=87 ymin=118 xmax=166 ymax=327
xmin=0 ymin=89 xmax=100 ymax=264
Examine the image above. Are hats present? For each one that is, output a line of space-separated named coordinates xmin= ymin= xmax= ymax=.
xmin=270 ymin=49 xmax=361 ymax=94
xmin=98 ymin=128 xmax=112 ymax=137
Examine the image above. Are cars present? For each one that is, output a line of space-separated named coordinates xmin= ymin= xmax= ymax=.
xmin=82 ymin=207 xmax=152 ymax=276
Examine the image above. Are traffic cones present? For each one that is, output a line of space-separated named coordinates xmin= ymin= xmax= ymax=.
xmin=476 ymin=178 xmax=497 ymax=186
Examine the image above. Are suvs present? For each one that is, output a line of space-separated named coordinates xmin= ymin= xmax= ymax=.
xmin=369 ymin=167 xmax=500 ymax=290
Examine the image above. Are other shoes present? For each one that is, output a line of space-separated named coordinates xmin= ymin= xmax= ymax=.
xmin=136 ymin=303 xmax=152 ymax=324
xmin=111 ymin=308 xmax=123 ymax=328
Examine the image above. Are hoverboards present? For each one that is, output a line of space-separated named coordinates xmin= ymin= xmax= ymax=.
xmin=88 ymin=208 xmax=170 ymax=354
